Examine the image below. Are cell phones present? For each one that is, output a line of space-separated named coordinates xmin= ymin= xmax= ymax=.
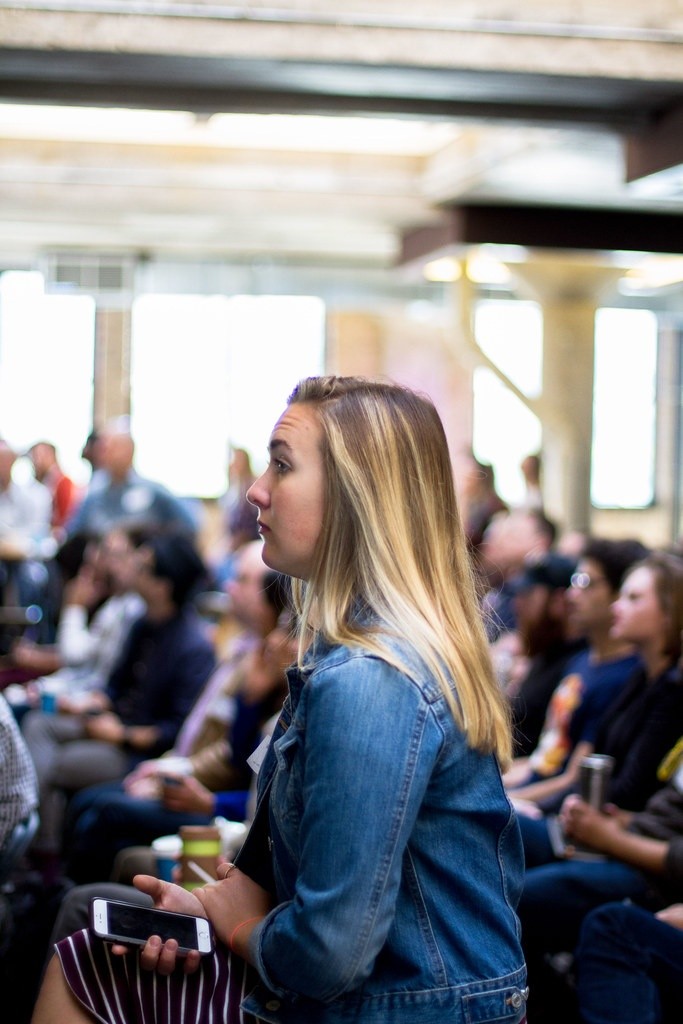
xmin=90 ymin=897 xmax=216 ymax=957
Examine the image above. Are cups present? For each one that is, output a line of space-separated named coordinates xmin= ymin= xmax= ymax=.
xmin=178 ymin=824 xmax=222 ymax=894
xmin=579 ymin=755 xmax=616 ymax=812
xmin=151 ymin=835 xmax=185 ymax=887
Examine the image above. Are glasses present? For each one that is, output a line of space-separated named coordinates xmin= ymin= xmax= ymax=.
xmin=569 ymin=572 xmax=610 ymax=591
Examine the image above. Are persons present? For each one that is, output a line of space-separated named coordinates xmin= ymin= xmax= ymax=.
xmin=0 ymin=370 xmax=682 ymax=1024
